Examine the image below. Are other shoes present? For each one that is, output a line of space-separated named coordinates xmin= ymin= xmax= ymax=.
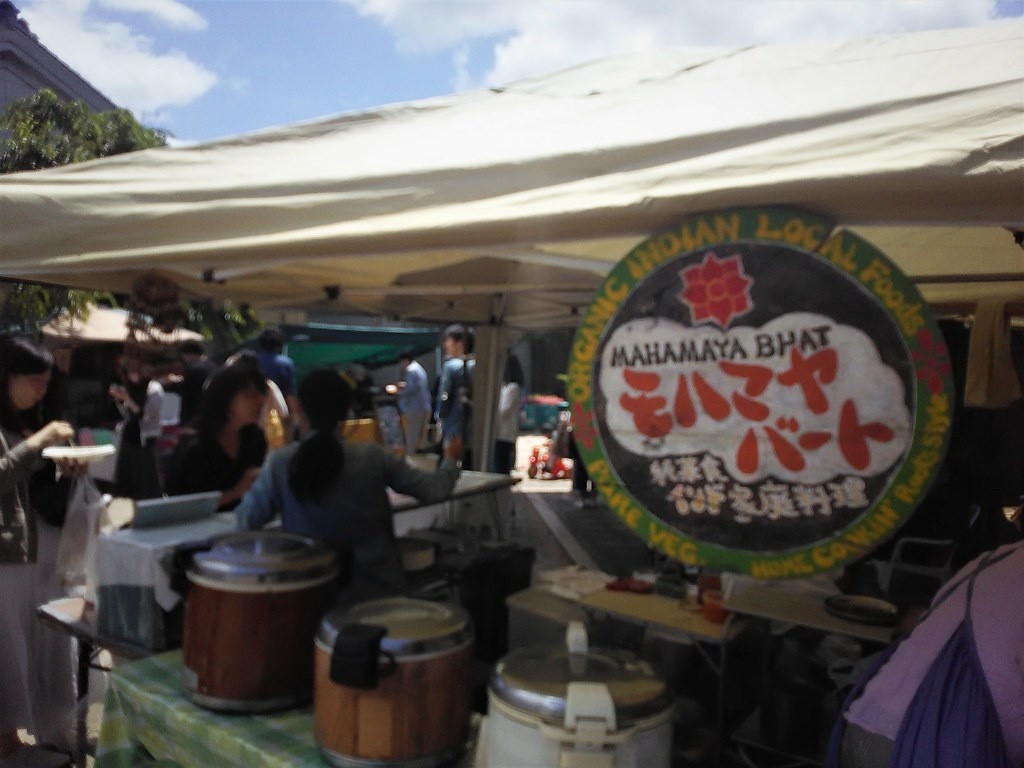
xmin=0 ymin=741 xmax=72 ymax=768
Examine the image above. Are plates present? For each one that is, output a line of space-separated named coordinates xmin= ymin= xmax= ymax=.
xmin=825 ymin=595 xmax=898 ymax=626
xmin=43 ymin=444 xmax=114 ymax=465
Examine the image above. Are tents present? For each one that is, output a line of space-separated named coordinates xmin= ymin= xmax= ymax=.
xmin=0 ymin=17 xmax=1024 ymax=485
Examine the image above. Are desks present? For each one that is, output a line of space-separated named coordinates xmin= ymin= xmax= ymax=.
xmin=37 ymin=471 xmax=898 ymax=768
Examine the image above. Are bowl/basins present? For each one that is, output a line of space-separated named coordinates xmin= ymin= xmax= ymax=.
xmin=702 ymin=589 xmax=726 ymax=623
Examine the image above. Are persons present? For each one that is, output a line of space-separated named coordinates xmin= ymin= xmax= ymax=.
xmin=1 ymin=336 xmax=91 ymax=767
xmin=107 ymin=323 xmax=526 ymax=510
xmin=544 ymin=411 xmax=598 ymax=507
xmin=235 ymin=369 xmax=466 ymax=604
xmin=824 ymin=539 xmax=1024 ymax=767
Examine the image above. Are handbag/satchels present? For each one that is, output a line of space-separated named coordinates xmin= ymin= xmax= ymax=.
xmin=53 ymin=472 xmax=108 ymax=587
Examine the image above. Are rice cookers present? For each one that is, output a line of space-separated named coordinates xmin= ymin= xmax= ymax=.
xmin=477 ymin=621 xmax=672 ymax=768
xmin=313 ymin=595 xmax=472 ymax=768
xmin=160 ymin=531 xmax=342 ymax=715
